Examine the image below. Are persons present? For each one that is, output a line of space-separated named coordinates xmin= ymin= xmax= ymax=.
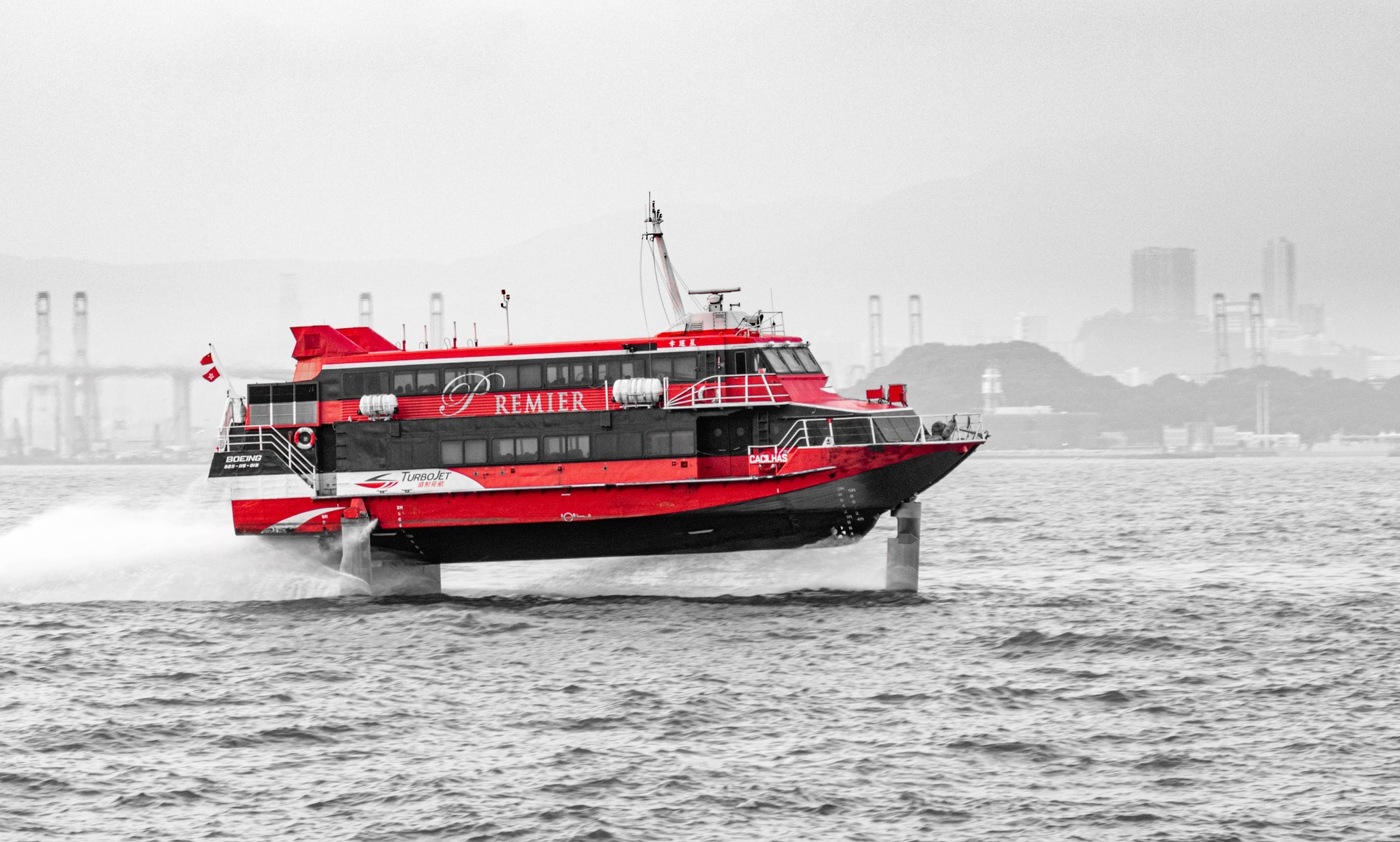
xmin=505 ymin=449 xmax=590 ymax=462
xmin=395 ymin=382 xmax=480 ymax=395
xmin=550 ymin=375 xmax=632 ymax=386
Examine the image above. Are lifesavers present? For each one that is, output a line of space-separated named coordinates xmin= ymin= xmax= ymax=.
xmin=698 ymin=384 xmax=721 ymax=403
xmin=758 ymin=452 xmax=776 ymax=473
xmin=293 ymin=427 xmax=316 ymax=450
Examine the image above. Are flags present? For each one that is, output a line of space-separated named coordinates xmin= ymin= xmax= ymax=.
xmin=202 ymin=366 xmax=221 ymax=382
xmin=200 ymin=352 xmax=213 ymax=366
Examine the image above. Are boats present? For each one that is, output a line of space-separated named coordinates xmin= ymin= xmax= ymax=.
xmin=205 ymin=189 xmax=992 ymax=568
